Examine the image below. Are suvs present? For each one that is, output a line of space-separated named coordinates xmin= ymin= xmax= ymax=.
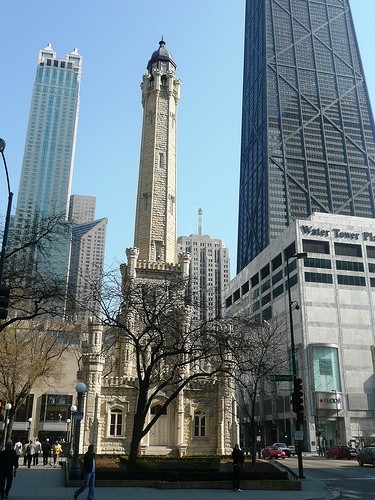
xmin=272 ymin=442 xmax=292 ymax=458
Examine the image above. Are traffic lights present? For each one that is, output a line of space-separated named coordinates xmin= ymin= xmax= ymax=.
xmin=294 ymin=377 xmax=304 ymax=412
xmin=0 ymin=284 xmax=11 ymax=319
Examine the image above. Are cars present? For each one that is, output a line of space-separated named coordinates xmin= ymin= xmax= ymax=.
xmin=325 ymin=445 xmax=363 ymax=460
xmin=256 ymin=445 xmax=286 ymax=459
xmin=287 ymin=445 xmax=298 ymax=456
xmin=356 ymin=446 xmax=375 ymax=467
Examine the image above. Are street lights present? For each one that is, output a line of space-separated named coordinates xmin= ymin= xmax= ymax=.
xmin=331 ymin=389 xmax=340 ymax=443
xmin=287 ymin=252 xmax=308 ymax=479
xmin=66 ymin=418 xmax=71 ymax=443
xmin=1 ymin=402 xmax=12 ymax=451
xmin=0 ymin=138 xmax=15 ymax=286
xmin=70 ymin=404 xmax=77 ymax=451
xmin=69 ymin=382 xmax=87 ymax=480
xmin=28 ymin=417 xmax=33 ymax=444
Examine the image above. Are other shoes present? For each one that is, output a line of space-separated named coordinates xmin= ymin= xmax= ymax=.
xmin=237 ymin=488 xmax=242 ymax=492
xmin=4 ymin=490 xmax=8 ymax=499
xmin=74 ymin=494 xmax=77 ymax=500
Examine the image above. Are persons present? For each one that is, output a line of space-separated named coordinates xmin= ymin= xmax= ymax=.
xmin=14 ymin=437 xmax=63 ymax=468
xmin=74 ymin=445 xmax=96 ymax=500
xmin=232 ymin=444 xmax=246 ymax=492
xmin=0 ymin=440 xmax=19 ymax=499
